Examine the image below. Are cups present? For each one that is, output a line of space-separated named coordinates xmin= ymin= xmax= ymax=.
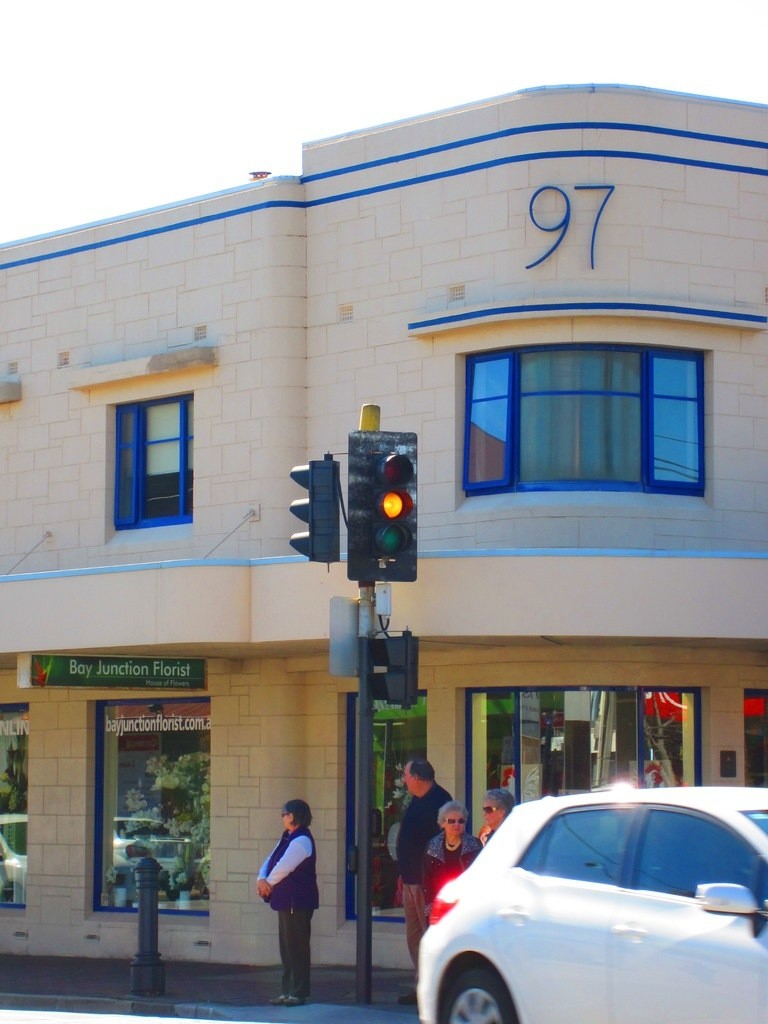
xmin=179 ymin=890 xmax=191 ymax=910
xmin=114 ymin=885 xmax=127 ymax=908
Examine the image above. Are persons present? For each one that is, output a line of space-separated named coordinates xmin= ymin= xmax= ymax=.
xmin=424 ymin=801 xmax=483 ymax=917
xmin=396 ymin=758 xmax=453 ymax=1004
xmin=256 ymin=799 xmax=319 ymax=1006
xmin=479 ymin=788 xmax=515 ymax=848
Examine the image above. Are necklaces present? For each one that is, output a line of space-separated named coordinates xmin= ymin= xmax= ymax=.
xmin=445 ymin=839 xmax=461 ymax=851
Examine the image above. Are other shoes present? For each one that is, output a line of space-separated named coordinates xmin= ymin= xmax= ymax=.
xmin=398 ymin=992 xmax=417 ymax=1005
xmin=269 ymin=994 xmax=289 ymax=1005
xmin=283 ymin=997 xmax=305 ymax=1005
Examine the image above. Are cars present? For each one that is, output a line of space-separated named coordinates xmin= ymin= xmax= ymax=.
xmin=416 ymin=787 xmax=768 ymax=1023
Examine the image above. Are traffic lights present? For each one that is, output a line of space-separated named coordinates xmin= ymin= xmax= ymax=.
xmin=286 ymin=460 xmax=341 ymax=562
xmin=347 ymin=433 xmax=417 ymax=584
xmin=375 ymin=634 xmax=418 ymax=709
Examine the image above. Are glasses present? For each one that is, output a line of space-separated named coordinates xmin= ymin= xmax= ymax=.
xmin=443 ymin=818 xmax=465 ymax=824
xmin=281 ymin=812 xmax=290 ymax=817
xmin=481 ymin=806 xmax=499 ymax=813
